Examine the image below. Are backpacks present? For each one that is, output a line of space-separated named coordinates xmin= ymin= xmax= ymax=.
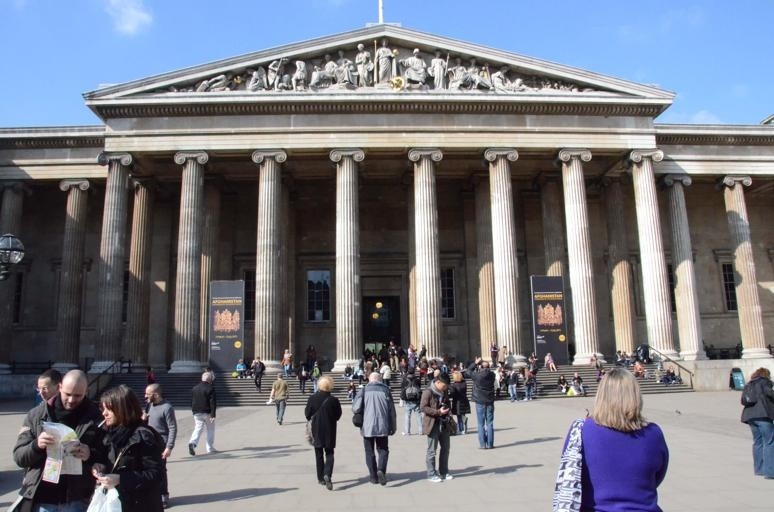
xmin=741 ymin=377 xmax=764 ymax=407
xmin=311 ymin=366 xmax=321 ymax=378
xmin=403 ymin=375 xmax=420 ymax=401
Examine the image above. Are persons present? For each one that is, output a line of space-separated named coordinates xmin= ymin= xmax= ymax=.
xmin=13 ymin=367 xmax=178 ymax=512
xmin=740 ymin=366 xmax=774 ymax=480
xmin=561 ymin=368 xmax=669 ymax=512
xmin=189 ymin=372 xmax=219 ymax=456
xmin=352 ymin=371 xmax=397 ymax=485
xmin=304 ymin=376 xmax=343 ymax=491
xmin=419 ymin=371 xmax=454 ymax=483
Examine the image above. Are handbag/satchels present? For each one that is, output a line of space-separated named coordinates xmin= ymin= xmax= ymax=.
xmin=351 ymin=408 xmax=363 ymax=427
xmin=304 ymin=418 xmax=314 ymax=445
xmin=302 ymin=370 xmax=309 ymax=377
xmin=554 ymin=419 xmax=588 ymax=511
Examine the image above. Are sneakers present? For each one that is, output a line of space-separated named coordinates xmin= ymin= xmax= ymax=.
xmin=419 ymin=432 xmax=423 ymax=435
xmin=317 ymin=480 xmax=325 ymax=484
xmin=208 ymin=450 xmax=218 ymax=453
xmin=188 ymin=443 xmax=195 ymax=456
xmin=427 ymin=477 xmax=441 ymax=483
xmin=369 ymin=478 xmax=377 ymax=484
xmin=439 ymin=473 xmax=453 ymax=480
xmin=377 ymin=469 xmax=386 ymax=486
xmin=323 ymin=474 xmax=332 ymax=490
xmin=401 ymin=432 xmax=411 ymax=436
